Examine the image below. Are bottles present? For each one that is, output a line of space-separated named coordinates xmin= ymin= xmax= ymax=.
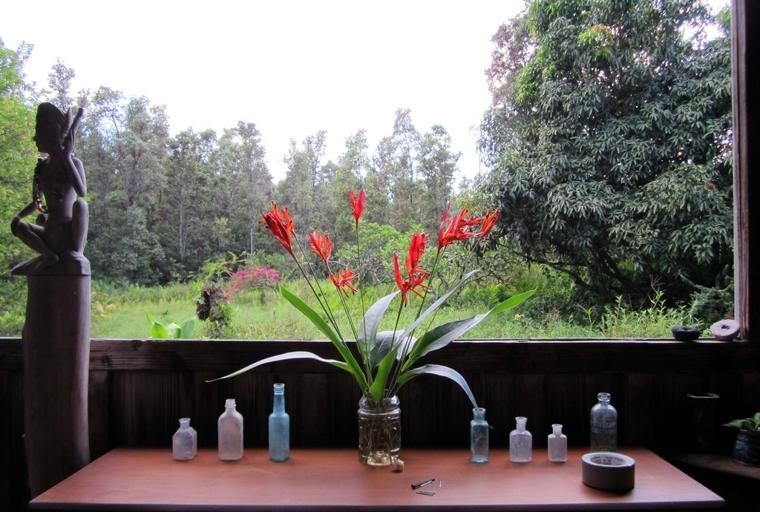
xmin=470 ymin=408 xmax=490 ymax=463
xmin=589 ymin=392 xmax=617 ymax=450
xmin=268 ymin=383 xmax=289 ymax=461
xmin=173 ymin=417 xmax=197 ymax=461
xmin=509 ymin=416 xmax=532 ymax=463
xmin=218 ymin=398 xmax=244 ymax=460
xmin=548 ymin=423 xmax=567 ymax=462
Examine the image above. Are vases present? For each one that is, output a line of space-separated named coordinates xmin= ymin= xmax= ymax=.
xmin=349 ymin=395 xmax=414 ymax=469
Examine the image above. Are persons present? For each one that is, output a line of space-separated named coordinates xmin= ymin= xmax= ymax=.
xmin=7 ymin=102 xmax=90 ymax=278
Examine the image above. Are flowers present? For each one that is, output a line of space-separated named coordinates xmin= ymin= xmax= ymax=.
xmin=201 ymin=186 xmax=522 ymax=408
